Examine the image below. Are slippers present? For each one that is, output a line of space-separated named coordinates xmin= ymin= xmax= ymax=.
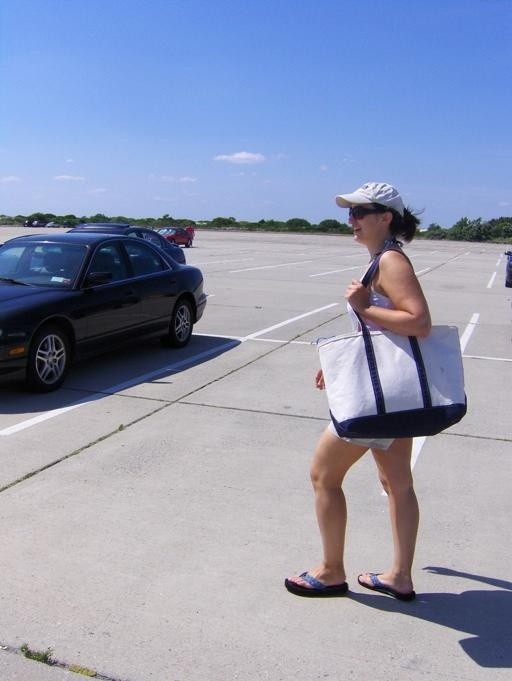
xmin=284 ymin=571 xmax=349 ymax=598
xmin=358 ymin=572 xmax=416 ymax=601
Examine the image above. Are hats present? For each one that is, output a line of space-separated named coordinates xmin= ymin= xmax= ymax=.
xmin=335 ymin=182 xmax=405 ymax=219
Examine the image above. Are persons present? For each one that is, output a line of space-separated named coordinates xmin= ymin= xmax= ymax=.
xmin=185 ymin=224 xmax=194 ymax=246
xmin=283 ymin=182 xmax=432 ymax=602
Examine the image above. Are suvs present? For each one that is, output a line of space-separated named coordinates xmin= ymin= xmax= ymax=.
xmin=63 ymin=220 xmax=71 ymax=228
xmin=23 ymin=219 xmax=33 ymax=228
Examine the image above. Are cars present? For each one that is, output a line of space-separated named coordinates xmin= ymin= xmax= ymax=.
xmin=503 ymin=249 xmax=512 ymax=289
xmin=33 ymin=221 xmax=46 ymax=228
xmin=69 ymin=223 xmax=187 ymax=273
xmin=46 ymin=221 xmax=60 ymax=228
xmin=0 ymin=231 xmax=210 ymax=395
xmin=155 ymin=225 xmax=194 ymax=249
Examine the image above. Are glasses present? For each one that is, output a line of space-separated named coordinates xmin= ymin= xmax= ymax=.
xmin=349 ymin=206 xmax=382 ymax=219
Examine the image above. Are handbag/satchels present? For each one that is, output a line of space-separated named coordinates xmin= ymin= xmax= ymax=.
xmin=316 ymin=242 xmax=469 ymax=438
